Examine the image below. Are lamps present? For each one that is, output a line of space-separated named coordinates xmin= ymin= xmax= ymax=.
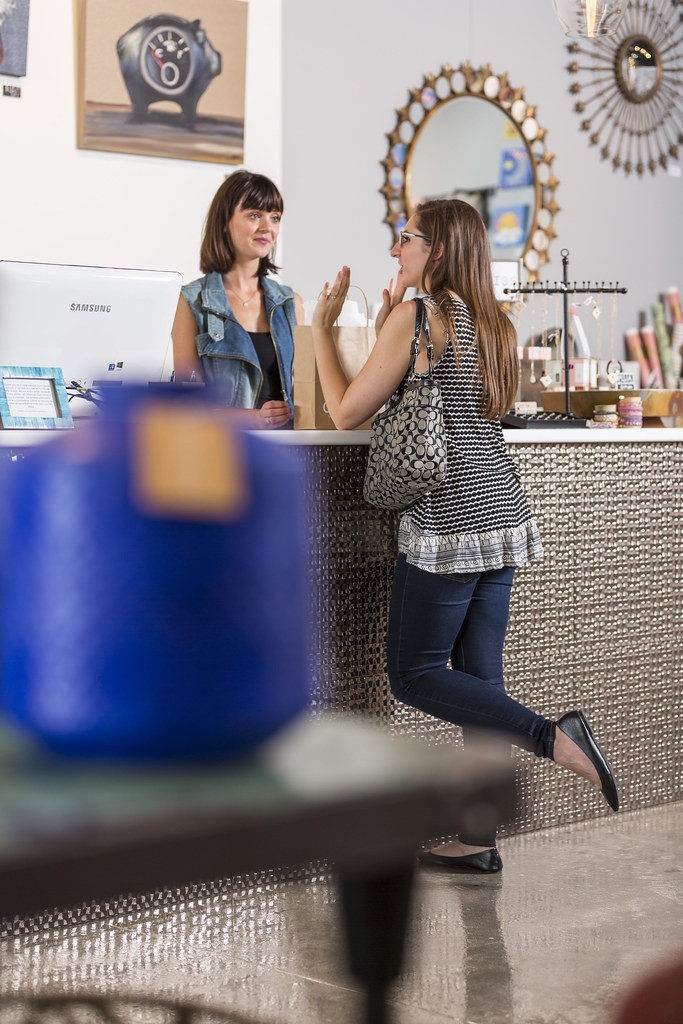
xmin=553 ymin=0 xmax=628 ymax=40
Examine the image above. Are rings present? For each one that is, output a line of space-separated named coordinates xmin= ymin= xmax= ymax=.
xmin=330 ymin=293 xmax=336 ymax=298
xmin=266 ymin=417 xmax=270 ymax=424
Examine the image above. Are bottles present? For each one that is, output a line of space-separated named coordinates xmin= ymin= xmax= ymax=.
xmin=1 ymin=382 xmax=316 ymax=765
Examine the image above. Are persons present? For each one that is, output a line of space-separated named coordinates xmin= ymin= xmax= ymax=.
xmin=311 ymin=199 xmax=619 ymax=871
xmin=172 ymin=172 xmax=305 ymax=429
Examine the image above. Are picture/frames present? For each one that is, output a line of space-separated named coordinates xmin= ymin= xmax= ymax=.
xmin=0 ymin=366 xmax=75 ymax=430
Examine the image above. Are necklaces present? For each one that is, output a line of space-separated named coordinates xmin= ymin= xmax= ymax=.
xmin=231 ymin=289 xmax=259 ymax=306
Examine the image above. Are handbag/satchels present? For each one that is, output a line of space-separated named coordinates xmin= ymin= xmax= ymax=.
xmin=363 ymin=299 xmax=447 ymax=510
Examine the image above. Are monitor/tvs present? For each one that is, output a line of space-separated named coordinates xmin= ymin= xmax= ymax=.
xmin=1 ymin=261 xmax=183 ymax=419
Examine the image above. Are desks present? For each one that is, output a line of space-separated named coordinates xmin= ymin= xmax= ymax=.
xmin=1 ymin=705 xmax=518 ymax=1024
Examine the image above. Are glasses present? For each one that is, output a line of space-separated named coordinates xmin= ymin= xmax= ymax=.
xmin=399 ymin=230 xmax=432 ymax=249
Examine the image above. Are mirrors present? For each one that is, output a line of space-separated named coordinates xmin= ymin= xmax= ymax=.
xmin=565 ymin=0 xmax=683 ymax=181
xmin=378 ymin=61 xmax=561 ymax=315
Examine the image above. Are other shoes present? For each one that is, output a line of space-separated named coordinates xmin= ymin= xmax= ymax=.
xmin=417 ymin=848 xmax=504 ymax=874
xmin=555 ymin=709 xmax=619 ymax=813
